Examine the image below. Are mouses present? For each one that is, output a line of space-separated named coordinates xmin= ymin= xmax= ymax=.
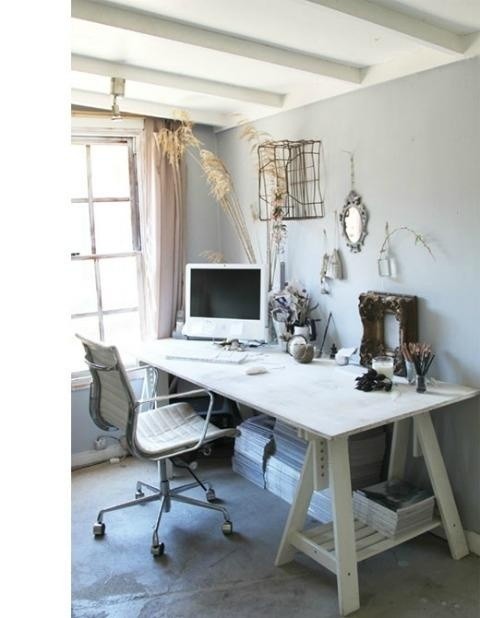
xmin=245 ymin=361 xmax=269 ymax=376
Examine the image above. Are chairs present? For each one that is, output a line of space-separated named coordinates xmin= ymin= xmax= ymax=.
xmin=74 ymin=333 xmax=242 ymax=558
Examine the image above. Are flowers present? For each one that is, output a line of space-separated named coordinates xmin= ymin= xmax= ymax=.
xmin=271 ymin=281 xmax=323 ymax=344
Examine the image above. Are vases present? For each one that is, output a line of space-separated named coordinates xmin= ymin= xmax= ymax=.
xmin=272 ymin=309 xmax=312 ymax=353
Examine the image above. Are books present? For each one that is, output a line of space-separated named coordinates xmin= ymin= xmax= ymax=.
xmin=231 ymin=415 xmax=435 ymax=538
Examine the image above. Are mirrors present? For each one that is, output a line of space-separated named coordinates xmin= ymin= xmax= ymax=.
xmin=339 ymin=189 xmax=367 ymax=253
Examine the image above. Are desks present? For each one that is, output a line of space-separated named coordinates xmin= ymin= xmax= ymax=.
xmin=135 ymin=337 xmax=479 ymax=614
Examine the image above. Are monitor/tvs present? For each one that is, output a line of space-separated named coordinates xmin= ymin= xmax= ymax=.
xmin=184 ymin=261 xmax=267 ymax=352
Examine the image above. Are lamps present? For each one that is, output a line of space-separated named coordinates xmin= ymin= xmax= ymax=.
xmin=110 ymin=77 xmax=124 ymax=118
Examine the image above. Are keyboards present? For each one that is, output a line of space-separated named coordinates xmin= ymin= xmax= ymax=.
xmin=166 ymin=342 xmax=250 ymax=365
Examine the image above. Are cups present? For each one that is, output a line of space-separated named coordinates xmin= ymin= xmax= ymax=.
xmin=372 ymin=357 xmax=395 ymax=386
xmin=293 ymin=345 xmax=316 ymax=364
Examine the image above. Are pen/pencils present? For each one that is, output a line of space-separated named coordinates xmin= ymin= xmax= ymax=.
xmin=400 ymin=342 xmax=433 ymax=362
xmin=315 ymin=312 xmax=332 ymax=358
xmin=410 ymin=351 xmax=436 ymax=393
